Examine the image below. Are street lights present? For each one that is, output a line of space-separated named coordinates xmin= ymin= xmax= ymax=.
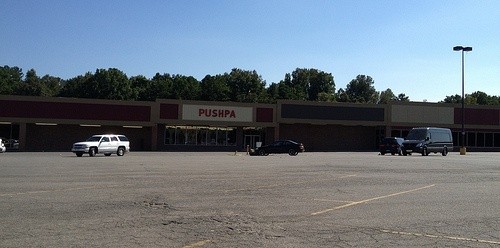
xmin=453 ymin=46 xmax=473 ymax=155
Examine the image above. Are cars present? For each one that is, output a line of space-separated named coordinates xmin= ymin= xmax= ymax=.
xmin=255 ymin=139 xmax=305 ymax=156
xmin=0 ymin=138 xmax=7 ymax=153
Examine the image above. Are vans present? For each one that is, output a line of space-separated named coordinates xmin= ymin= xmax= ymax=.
xmin=400 ymin=127 xmax=454 ymax=157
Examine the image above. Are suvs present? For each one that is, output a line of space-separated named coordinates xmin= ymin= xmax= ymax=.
xmin=379 ymin=137 xmax=406 ymax=157
xmin=72 ymin=134 xmax=130 ymax=157
